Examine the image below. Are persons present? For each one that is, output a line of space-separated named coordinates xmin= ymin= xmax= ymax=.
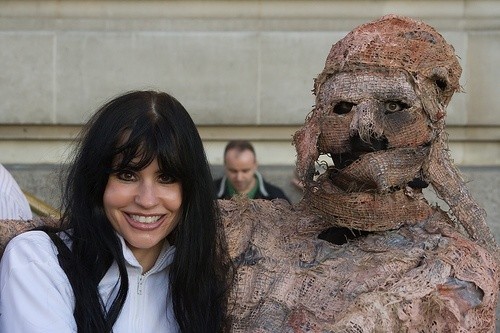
xmin=212 ymin=139 xmax=291 ymax=205
xmin=0 ymin=90 xmax=237 ymax=333
